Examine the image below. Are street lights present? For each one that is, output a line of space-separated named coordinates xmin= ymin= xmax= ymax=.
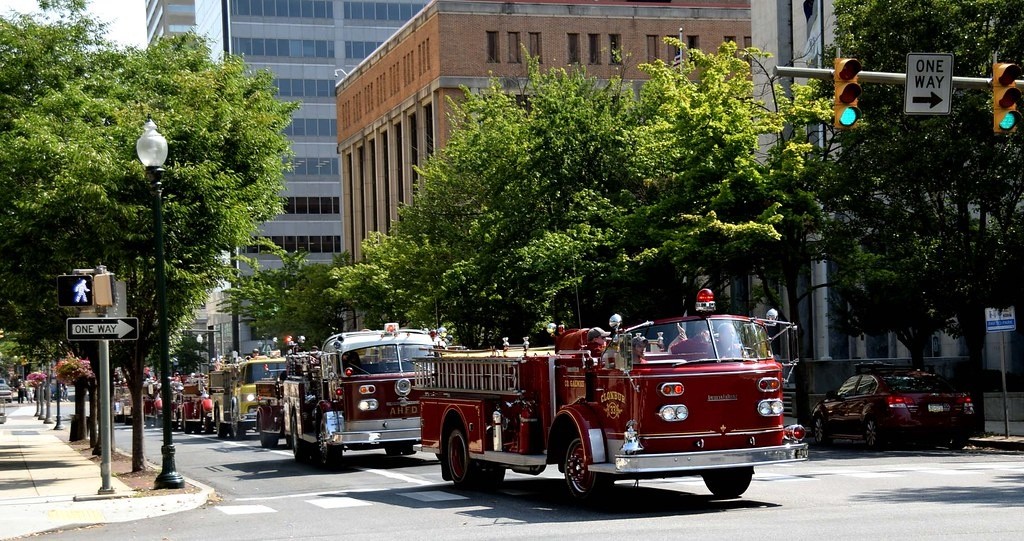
xmin=136 ymin=117 xmax=185 ymax=490
xmin=196 ymin=333 xmax=203 ymax=373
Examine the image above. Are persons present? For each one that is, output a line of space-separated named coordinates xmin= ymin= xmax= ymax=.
xmin=579 ymin=327 xmax=611 ymax=358
xmin=63 ymin=384 xmax=68 ymax=402
xmin=632 ymin=337 xmax=648 ymax=363
xmin=347 ymin=352 xmax=361 ymax=375
xmin=18 ymin=381 xmax=25 ymax=403
xmin=707 ymin=323 xmax=745 ymax=359
xmin=26 ymin=386 xmax=35 ymax=403
xmin=668 ymin=321 xmax=711 ymax=354
xmin=247 ymin=349 xmax=259 ymax=360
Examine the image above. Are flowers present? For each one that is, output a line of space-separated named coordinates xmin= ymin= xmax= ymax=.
xmin=27 ymin=372 xmax=47 ymax=386
xmin=52 ymin=351 xmax=96 ymax=385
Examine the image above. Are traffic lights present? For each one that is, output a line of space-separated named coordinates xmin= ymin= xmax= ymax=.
xmin=96 ymin=272 xmax=118 ymax=308
xmin=992 ymin=61 xmax=1023 ymax=135
xmin=833 ymin=56 xmax=862 ymax=130
xmin=56 ymin=274 xmax=94 ymax=309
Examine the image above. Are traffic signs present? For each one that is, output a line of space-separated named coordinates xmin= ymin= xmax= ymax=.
xmin=66 ymin=316 xmax=139 ymax=341
xmin=904 ymin=53 xmax=954 ymax=115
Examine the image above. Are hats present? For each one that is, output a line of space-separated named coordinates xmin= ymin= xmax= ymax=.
xmin=587 ymin=326 xmax=612 ymax=340
xmin=253 ymin=349 xmax=258 ymax=352
xmin=631 ymin=336 xmax=648 ymax=347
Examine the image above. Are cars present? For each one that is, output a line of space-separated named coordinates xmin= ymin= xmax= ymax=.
xmin=809 ymin=363 xmax=977 ymax=454
xmin=0 ymin=377 xmax=14 ymax=404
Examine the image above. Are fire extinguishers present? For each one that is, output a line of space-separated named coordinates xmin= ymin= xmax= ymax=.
xmin=490 ymin=405 xmax=506 ymax=452
xmin=519 ymin=401 xmax=537 ymax=454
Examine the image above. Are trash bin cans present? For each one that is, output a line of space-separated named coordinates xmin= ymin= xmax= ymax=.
xmin=68 ymin=414 xmax=91 ymax=442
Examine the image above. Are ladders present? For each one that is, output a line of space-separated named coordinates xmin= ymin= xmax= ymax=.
xmin=410 ymin=357 xmax=521 ymax=395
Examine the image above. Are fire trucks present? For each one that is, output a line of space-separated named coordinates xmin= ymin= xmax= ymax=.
xmin=409 ymin=285 xmax=808 ymax=509
xmin=111 ymin=348 xmax=286 ymax=442
xmin=282 ymin=322 xmax=454 ymax=474
